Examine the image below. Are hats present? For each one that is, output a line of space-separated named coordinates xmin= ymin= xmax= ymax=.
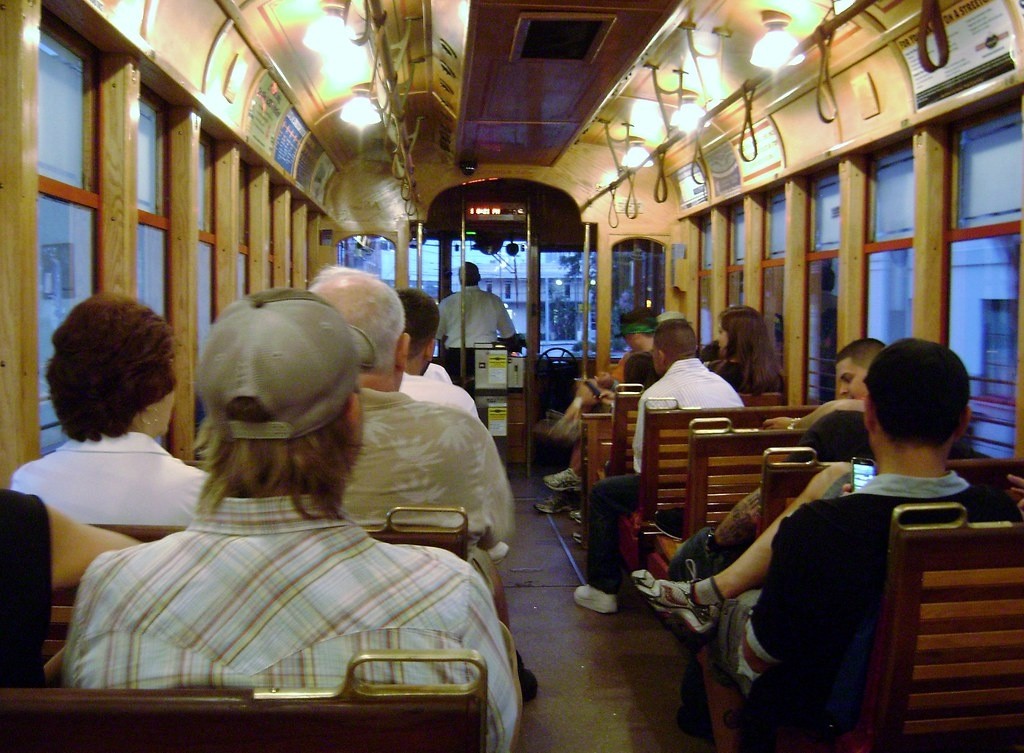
xmin=202 ymin=287 xmax=375 ymax=440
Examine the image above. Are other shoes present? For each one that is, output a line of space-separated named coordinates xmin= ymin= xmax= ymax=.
xmin=572 ymin=531 xmax=582 ymax=542
xmin=570 ymin=508 xmax=582 ymax=524
xmin=653 ymin=506 xmax=688 ymax=540
xmin=514 ymin=650 xmax=537 ymax=704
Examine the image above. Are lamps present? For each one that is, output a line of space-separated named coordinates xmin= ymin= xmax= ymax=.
xmin=749 ymin=8 xmax=806 ymax=67
xmin=666 ymin=91 xmax=708 ymax=132
xmin=622 ymin=132 xmax=653 ymax=170
xmin=341 ymin=81 xmax=381 ymax=128
xmin=303 ymin=2 xmax=356 ymax=56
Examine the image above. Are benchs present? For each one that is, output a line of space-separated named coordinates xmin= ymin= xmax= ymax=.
xmin=0 ymin=498 xmax=488 ymax=753
xmin=569 ymin=390 xmax=1024 ymax=753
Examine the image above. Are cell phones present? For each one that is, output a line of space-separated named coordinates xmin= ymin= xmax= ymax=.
xmin=851 ymin=457 xmax=877 ymax=493
xmin=585 ymin=381 xmax=603 ymax=399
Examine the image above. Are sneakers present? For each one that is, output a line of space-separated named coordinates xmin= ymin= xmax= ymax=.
xmin=533 ymin=494 xmax=578 ymax=513
xmin=574 ymin=585 xmax=617 ymax=614
xmin=632 ymin=569 xmax=720 ymax=645
xmin=542 ymin=469 xmax=583 ymax=492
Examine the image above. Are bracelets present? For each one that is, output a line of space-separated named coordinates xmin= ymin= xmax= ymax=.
xmin=787 ymin=417 xmax=801 ymax=430
xmin=610 ymin=380 xmax=618 ymax=391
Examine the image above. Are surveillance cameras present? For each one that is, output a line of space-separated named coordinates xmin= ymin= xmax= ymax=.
xmin=458 ymin=160 xmax=478 ymax=176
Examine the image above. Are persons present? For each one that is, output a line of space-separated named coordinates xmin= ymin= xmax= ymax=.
xmin=397 ymin=290 xmax=479 ymax=418
xmin=0 ymin=489 xmax=143 ymax=689
xmin=11 ymin=290 xmax=208 ymax=526
xmin=716 ymin=306 xmax=785 ymax=395
xmin=311 ymin=267 xmax=537 ymax=703
xmin=573 ymin=319 xmax=746 ymax=613
xmin=62 ymin=290 xmax=518 ymax=753
xmin=534 ymin=306 xmax=721 ymax=544
xmin=435 ymin=261 xmax=515 ymax=376
xmin=625 ymin=337 xmax=1024 ymax=753
